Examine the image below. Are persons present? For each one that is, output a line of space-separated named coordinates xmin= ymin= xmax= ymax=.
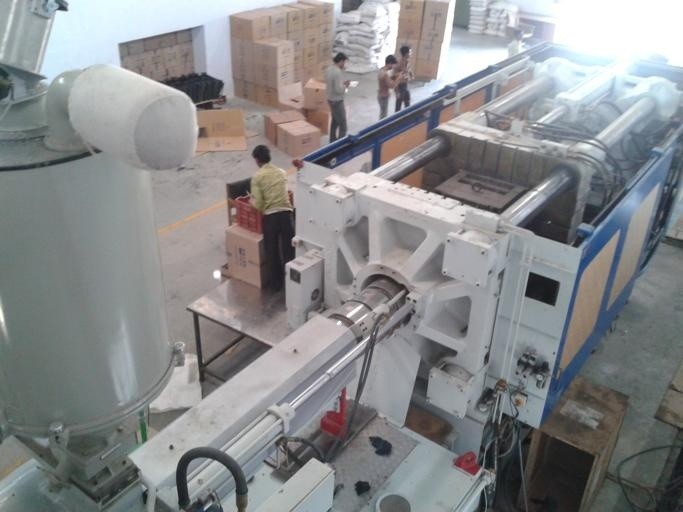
xmin=392 ymin=46 xmax=415 ymax=113
xmin=506 ymin=30 xmax=524 ymax=57
xmin=375 ymin=55 xmax=403 ymax=119
xmin=248 ymin=144 xmax=296 ymax=294
xmin=325 ymin=53 xmax=352 ymax=143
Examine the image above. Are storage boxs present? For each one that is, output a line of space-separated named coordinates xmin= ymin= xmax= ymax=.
xmin=393 ymin=0 xmax=455 ymax=80
xmin=227 ymin=1 xmax=333 ymax=113
xmin=226 ymin=222 xmax=283 ymax=290
xmin=307 ymin=110 xmax=333 ymax=135
xmin=262 ymin=110 xmax=303 ymax=143
xmin=277 ymin=120 xmax=322 ymax=158
xmin=236 ymin=190 xmax=294 ymax=233
xmin=515 ymin=375 xmax=631 ymax=510
